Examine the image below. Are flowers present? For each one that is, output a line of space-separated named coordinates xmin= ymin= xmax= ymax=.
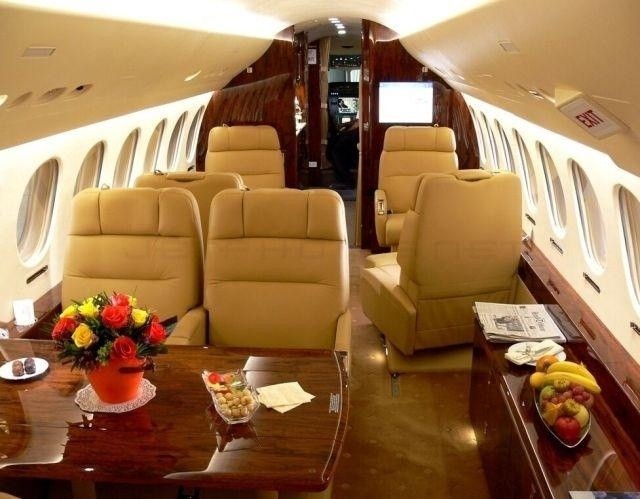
xmin=54 ymin=287 xmax=179 ymax=371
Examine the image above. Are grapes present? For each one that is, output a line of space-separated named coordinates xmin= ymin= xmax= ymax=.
xmin=551 ymin=385 xmax=591 ymax=404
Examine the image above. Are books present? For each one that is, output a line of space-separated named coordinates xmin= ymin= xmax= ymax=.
xmin=472 ymin=301 xmax=567 ymax=342
xmin=569 ymin=489 xmax=640 ymax=499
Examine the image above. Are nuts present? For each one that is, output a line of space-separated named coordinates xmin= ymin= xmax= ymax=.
xmin=214 ymin=390 xmax=254 ymax=420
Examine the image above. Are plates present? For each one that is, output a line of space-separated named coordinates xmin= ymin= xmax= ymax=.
xmin=509 ymin=342 xmax=567 ymax=367
xmin=533 ymin=375 xmax=592 ymax=448
xmin=0 ymin=356 xmax=50 ymax=381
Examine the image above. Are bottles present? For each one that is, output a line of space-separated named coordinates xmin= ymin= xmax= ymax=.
xmin=295 ymin=96 xmax=302 ymax=120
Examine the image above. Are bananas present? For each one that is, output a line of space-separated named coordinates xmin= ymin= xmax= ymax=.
xmin=546 ymin=361 xmax=602 ymax=396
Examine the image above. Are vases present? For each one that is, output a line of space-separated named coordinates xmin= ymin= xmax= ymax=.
xmin=80 ymin=362 xmax=145 ymax=402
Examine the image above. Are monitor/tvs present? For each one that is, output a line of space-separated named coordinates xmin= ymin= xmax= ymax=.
xmin=379 ymin=81 xmax=433 ymax=124
xmin=338 ymin=96 xmax=359 ymax=115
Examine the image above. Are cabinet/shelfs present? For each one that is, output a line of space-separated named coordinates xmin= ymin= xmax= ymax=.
xmin=462 ymin=308 xmax=638 ymax=498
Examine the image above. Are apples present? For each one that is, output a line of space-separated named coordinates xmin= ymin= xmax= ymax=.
xmin=555 ymin=416 xmax=582 ymax=445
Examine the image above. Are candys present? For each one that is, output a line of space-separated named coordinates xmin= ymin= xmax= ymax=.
xmin=11 ymin=358 xmax=37 ymax=376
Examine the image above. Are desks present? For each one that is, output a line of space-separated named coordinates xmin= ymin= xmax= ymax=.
xmin=0 ymin=336 xmax=349 ymax=499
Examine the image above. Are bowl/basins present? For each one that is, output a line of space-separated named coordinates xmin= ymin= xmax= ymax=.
xmin=198 ymin=368 xmax=261 ymax=425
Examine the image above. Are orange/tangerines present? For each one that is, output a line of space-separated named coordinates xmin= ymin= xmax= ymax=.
xmin=535 ymin=356 xmax=557 ymax=372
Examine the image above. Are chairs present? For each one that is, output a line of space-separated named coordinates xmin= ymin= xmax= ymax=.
xmin=163 ymin=189 xmax=353 ymax=380
xmin=357 ymin=169 xmax=525 ymax=358
xmin=201 ymin=122 xmax=286 ymax=190
xmin=371 ymin=125 xmax=459 ymax=253
xmin=135 ymin=171 xmax=244 ymax=247
xmin=53 ymin=186 xmax=203 ymax=350
